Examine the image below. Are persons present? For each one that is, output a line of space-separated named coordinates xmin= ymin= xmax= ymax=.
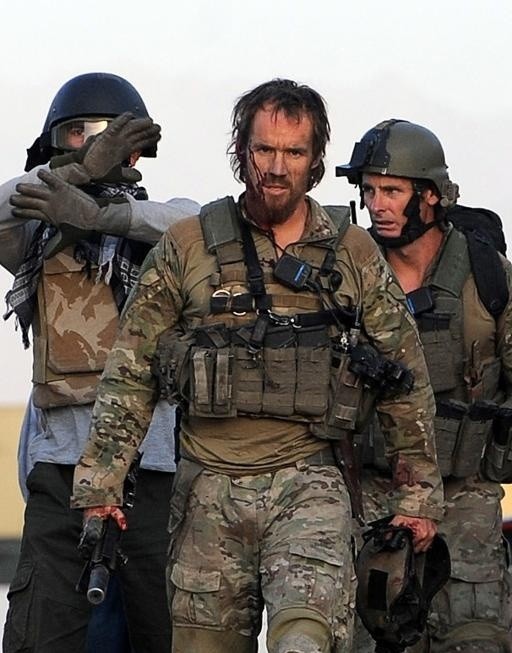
xmin=69 ymin=77 xmax=446 ymax=653
xmin=331 ymin=116 xmax=511 ymax=653
xmin=0 ymin=70 xmax=200 ymax=652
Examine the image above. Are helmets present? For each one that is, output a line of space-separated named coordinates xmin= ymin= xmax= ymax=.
xmin=40 ymin=65 xmax=161 ymax=164
xmin=334 ymin=116 xmax=462 ymax=205
xmin=355 ymin=520 xmax=451 ymax=644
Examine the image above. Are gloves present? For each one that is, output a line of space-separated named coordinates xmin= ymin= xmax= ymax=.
xmin=49 ymin=111 xmax=161 ymax=182
xmin=9 ymin=168 xmax=132 ymax=261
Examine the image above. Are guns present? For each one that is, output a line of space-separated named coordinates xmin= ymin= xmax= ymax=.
xmin=76 ymin=451 xmax=142 ymax=605
